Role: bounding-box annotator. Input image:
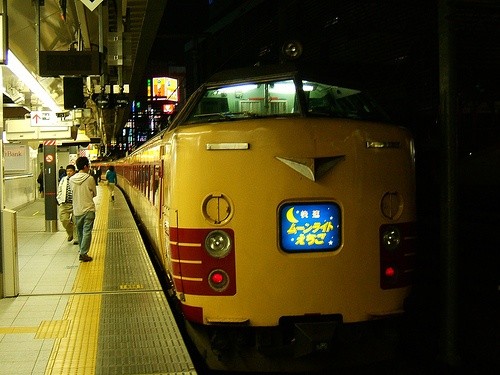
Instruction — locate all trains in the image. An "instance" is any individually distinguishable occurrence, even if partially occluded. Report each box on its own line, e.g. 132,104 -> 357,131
91,40 -> 418,361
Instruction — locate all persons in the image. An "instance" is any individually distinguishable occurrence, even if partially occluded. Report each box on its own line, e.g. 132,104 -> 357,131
59,166 -> 67,181
87,165 -> 102,185
105,166 -> 117,201
57,165 -> 80,245
37,169 -> 44,193
70,157 -> 97,262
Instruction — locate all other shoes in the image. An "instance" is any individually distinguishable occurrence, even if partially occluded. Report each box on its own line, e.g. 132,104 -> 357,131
68,236 -> 73,241
78,254 -> 92,261
72,241 -> 79,245
112,196 -> 114,200
